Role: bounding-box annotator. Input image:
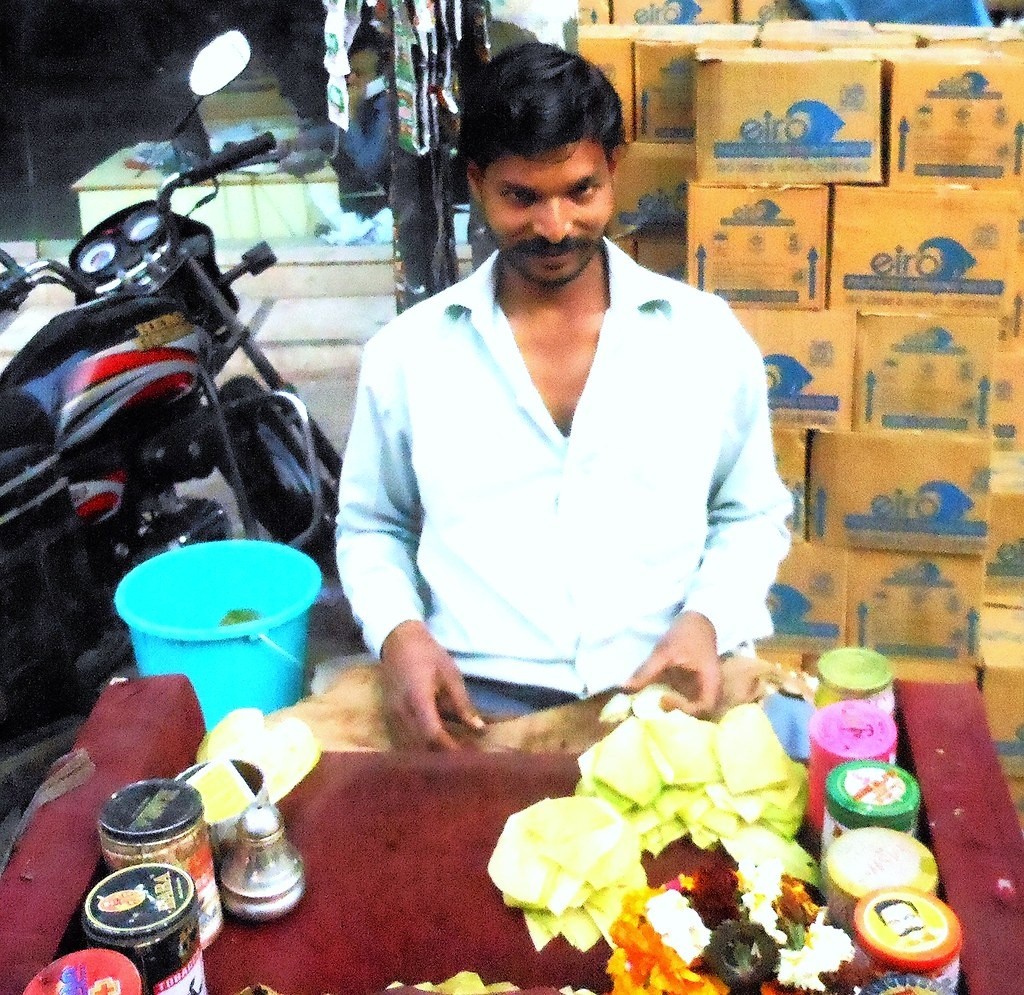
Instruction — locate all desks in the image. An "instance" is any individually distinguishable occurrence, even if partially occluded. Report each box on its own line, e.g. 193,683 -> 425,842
0,681 -> 1024,995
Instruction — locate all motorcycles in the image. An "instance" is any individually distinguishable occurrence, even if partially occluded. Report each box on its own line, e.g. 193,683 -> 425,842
0,25 -> 360,770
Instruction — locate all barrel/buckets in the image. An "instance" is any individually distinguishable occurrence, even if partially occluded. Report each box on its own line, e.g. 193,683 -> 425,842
112,539 -> 322,739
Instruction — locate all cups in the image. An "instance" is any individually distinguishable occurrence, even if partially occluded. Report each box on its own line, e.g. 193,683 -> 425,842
171,757 -> 270,871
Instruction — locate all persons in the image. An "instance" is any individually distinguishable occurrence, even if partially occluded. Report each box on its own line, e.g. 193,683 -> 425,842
334,42 -> 793,755
276,39 -> 390,185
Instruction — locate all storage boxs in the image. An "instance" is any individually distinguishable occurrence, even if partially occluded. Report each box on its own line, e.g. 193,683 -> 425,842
574,0 -> 1024,836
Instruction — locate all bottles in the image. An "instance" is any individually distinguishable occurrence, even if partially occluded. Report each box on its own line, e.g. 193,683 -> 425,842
805,645 -> 962,995
19,778 -> 222,995
